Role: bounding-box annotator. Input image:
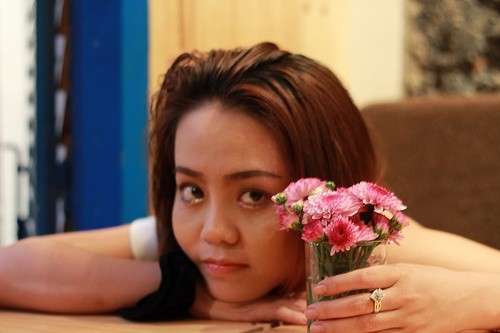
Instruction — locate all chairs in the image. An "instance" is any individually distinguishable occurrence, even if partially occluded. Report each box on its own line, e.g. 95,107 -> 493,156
359,94 -> 500,252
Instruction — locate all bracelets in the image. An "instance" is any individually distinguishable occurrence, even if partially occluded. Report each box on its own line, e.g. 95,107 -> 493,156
120,250 -> 196,322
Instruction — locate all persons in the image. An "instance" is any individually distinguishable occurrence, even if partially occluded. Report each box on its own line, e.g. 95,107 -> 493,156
0,42 -> 500,333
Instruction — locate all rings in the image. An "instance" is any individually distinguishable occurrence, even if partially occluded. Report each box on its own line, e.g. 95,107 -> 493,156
370,289 -> 385,314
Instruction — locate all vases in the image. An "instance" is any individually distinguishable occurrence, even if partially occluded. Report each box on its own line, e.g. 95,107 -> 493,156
306,236 -> 387,333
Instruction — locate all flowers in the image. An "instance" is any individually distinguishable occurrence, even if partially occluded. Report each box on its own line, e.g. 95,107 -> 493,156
271,177 -> 411,319
